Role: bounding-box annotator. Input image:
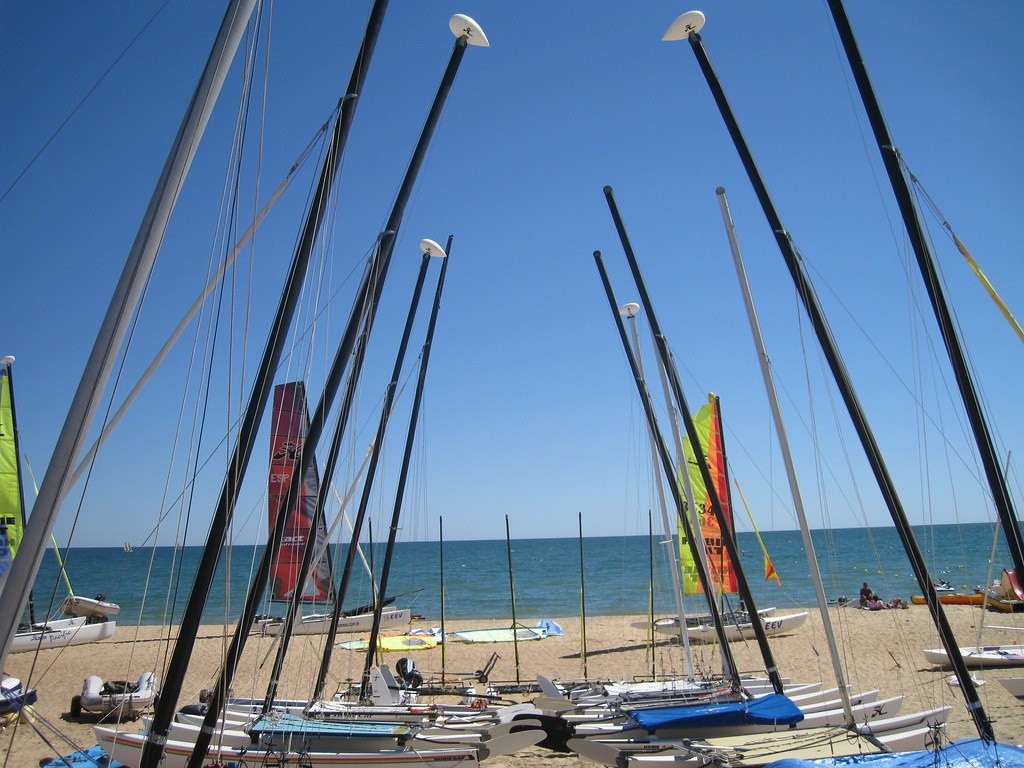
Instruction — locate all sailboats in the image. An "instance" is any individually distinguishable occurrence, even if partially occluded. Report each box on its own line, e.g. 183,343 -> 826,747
536,0 -> 1024,768
1,0 -> 566,768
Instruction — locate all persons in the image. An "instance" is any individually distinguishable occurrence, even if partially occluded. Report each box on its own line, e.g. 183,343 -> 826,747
860,583 -> 889,610
